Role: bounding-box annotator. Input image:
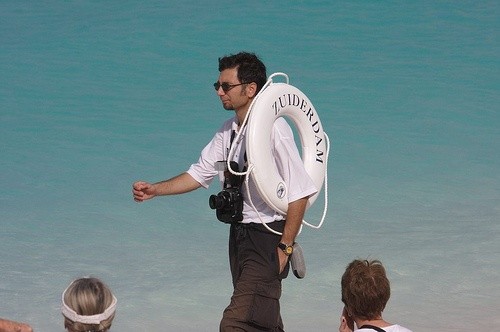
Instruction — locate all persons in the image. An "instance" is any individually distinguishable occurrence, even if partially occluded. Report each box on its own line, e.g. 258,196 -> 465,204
131,49 -> 319,331
337,257 -> 414,332
57,276 -> 117,332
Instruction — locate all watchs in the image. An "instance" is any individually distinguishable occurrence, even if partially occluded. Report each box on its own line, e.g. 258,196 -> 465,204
277,242 -> 294,256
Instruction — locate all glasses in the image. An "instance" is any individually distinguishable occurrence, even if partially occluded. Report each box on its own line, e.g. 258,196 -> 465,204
214,81 -> 252,92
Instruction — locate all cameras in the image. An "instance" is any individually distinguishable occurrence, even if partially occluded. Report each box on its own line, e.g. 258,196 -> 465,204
209,161 -> 243,223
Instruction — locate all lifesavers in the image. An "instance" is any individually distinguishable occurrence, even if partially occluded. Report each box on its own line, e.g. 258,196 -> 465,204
246,82 -> 326,217
